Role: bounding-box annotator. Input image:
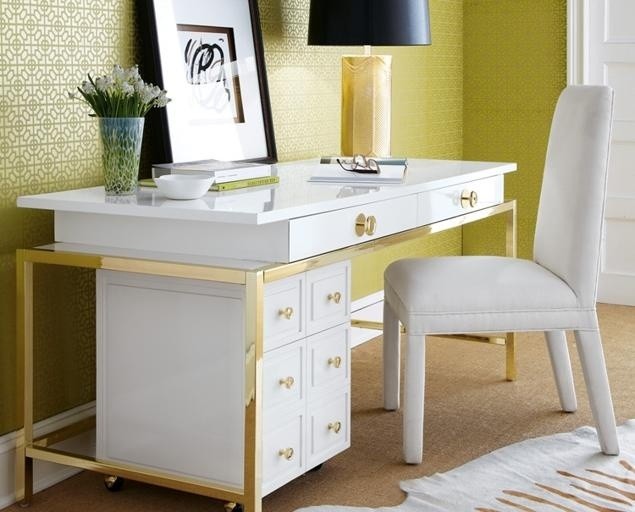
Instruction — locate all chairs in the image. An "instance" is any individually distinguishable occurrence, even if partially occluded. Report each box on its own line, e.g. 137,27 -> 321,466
382,84 -> 620,466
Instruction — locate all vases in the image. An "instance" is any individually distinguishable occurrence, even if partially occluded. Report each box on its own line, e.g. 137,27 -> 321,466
97,117 -> 146,196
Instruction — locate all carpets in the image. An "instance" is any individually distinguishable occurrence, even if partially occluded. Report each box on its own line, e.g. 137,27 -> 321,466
292,417 -> 635,512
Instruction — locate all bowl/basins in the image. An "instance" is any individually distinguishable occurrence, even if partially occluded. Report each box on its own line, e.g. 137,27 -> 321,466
153,174 -> 216,200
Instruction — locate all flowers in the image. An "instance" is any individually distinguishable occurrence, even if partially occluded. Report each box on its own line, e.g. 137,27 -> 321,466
68,63 -> 171,193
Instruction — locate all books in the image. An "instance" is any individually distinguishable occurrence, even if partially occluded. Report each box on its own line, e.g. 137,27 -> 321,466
308,157 -> 408,185
138,158 -> 280,191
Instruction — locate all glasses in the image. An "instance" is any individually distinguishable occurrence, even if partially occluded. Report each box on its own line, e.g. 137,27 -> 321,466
337,153 -> 380,173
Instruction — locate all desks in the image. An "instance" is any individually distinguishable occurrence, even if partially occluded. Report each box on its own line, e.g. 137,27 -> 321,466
14,158 -> 518,512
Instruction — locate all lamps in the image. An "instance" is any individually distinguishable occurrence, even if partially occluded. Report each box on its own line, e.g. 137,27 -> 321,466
306,0 -> 432,158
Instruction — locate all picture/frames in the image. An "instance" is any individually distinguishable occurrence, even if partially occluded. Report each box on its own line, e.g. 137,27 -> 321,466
135,0 -> 278,164
